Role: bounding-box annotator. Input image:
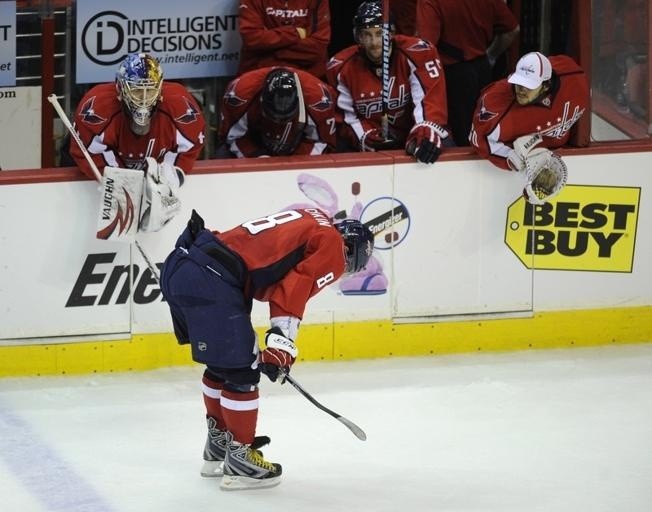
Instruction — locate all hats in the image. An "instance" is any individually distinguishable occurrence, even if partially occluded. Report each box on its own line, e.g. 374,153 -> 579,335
508,51 -> 552,90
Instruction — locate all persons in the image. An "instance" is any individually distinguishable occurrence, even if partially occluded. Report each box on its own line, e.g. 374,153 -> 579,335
323,1 -> 453,164
61,52 -> 207,232
159,207 -> 374,490
236,0 -> 331,78
468,52 -> 592,172
413,0 -> 521,147
215,65 -> 338,159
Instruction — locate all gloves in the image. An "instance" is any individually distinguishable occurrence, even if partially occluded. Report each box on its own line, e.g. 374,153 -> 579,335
405,121 -> 448,164
362,129 -> 402,152
259,333 -> 297,381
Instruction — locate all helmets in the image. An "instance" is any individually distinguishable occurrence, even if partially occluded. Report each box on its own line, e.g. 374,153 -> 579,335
334,219 -> 374,273
260,68 -> 300,121
352,2 -> 391,45
115,53 -> 164,137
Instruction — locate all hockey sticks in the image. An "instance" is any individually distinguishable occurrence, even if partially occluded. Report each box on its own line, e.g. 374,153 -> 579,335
279,71 -> 307,156
286,374 -> 366,440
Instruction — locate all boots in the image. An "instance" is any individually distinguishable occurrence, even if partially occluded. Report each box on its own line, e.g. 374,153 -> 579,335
220,432 -> 282,479
203,417 -> 262,462
